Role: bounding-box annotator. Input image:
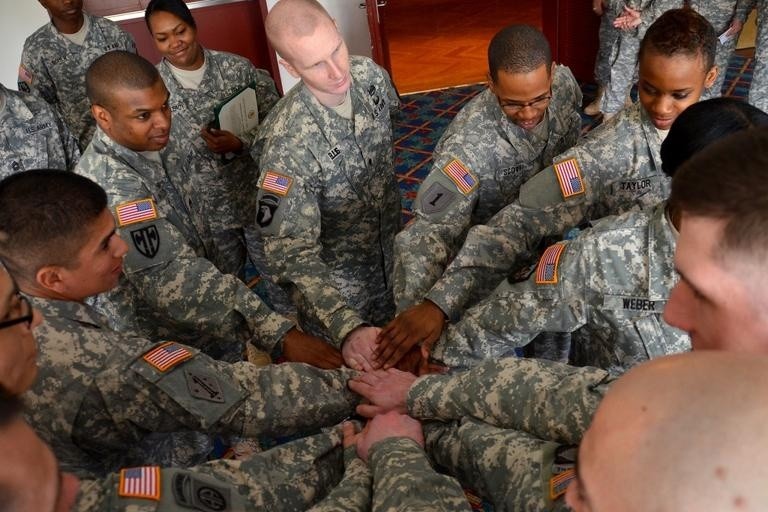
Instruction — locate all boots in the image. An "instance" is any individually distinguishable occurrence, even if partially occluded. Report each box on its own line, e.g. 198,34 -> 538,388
584,85 -> 633,123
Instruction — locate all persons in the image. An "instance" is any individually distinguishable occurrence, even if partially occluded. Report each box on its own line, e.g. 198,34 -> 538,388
0,0 -> 768,512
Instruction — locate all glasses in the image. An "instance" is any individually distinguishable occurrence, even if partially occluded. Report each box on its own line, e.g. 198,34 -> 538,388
0,258 -> 33,329
495,84 -> 552,110
